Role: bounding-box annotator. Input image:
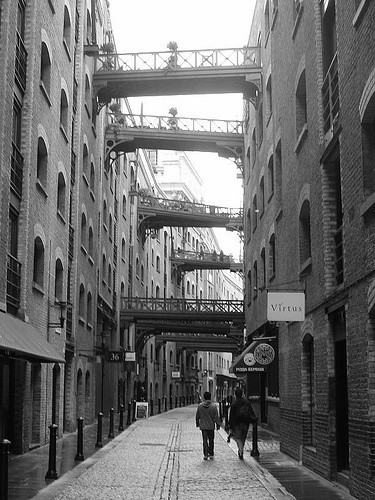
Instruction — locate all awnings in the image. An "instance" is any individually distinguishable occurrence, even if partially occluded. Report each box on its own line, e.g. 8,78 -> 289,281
229,336 -> 277,373
0,310 -> 65,363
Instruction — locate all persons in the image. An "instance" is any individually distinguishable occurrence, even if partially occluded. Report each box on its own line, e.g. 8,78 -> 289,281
229,388 -> 257,459
195,392 -> 219,460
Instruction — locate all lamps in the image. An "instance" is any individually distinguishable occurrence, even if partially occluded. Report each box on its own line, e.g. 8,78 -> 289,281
109,150 -> 118,160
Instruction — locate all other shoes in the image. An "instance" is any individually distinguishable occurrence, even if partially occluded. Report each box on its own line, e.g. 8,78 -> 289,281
239,451 -> 244,459
203,456 -> 208,460
210,456 -> 214,460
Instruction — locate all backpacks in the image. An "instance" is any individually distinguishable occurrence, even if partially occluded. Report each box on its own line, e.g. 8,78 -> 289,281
235,401 -> 251,422
